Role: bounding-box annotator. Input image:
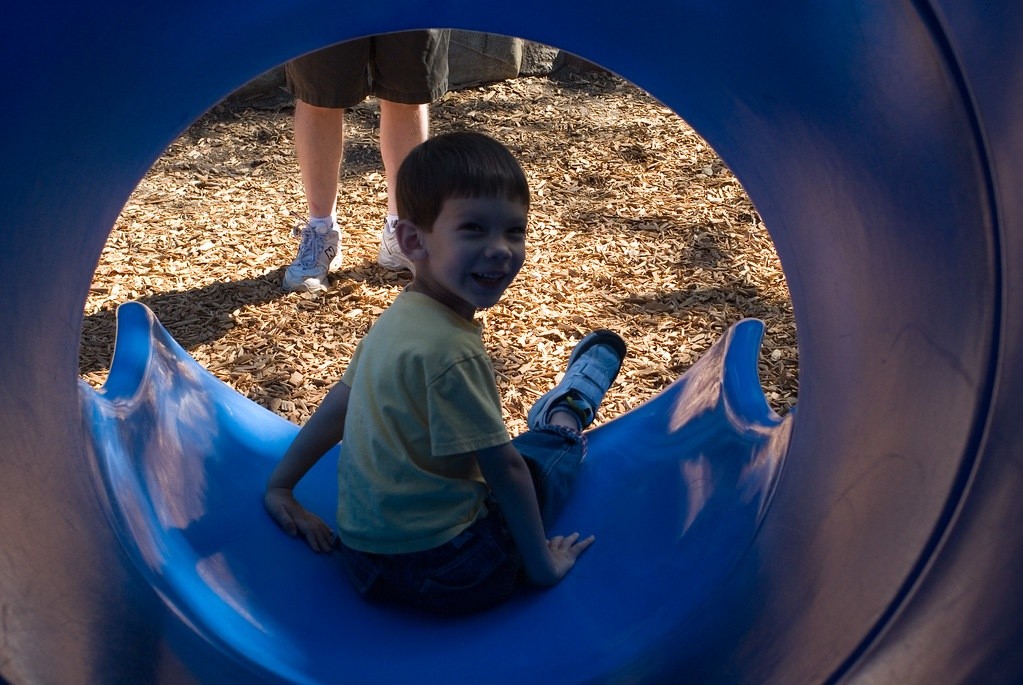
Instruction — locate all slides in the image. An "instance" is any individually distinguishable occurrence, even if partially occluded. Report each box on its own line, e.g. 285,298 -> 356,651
0,0 -> 1023,684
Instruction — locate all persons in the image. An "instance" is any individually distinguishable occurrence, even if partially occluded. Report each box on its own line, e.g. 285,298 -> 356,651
281,27 -> 451,296
263,132 -> 627,621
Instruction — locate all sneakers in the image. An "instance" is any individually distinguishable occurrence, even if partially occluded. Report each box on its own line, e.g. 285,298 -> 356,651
527,330 -> 626,432
378,218 -> 416,278
283,210 -> 342,293
